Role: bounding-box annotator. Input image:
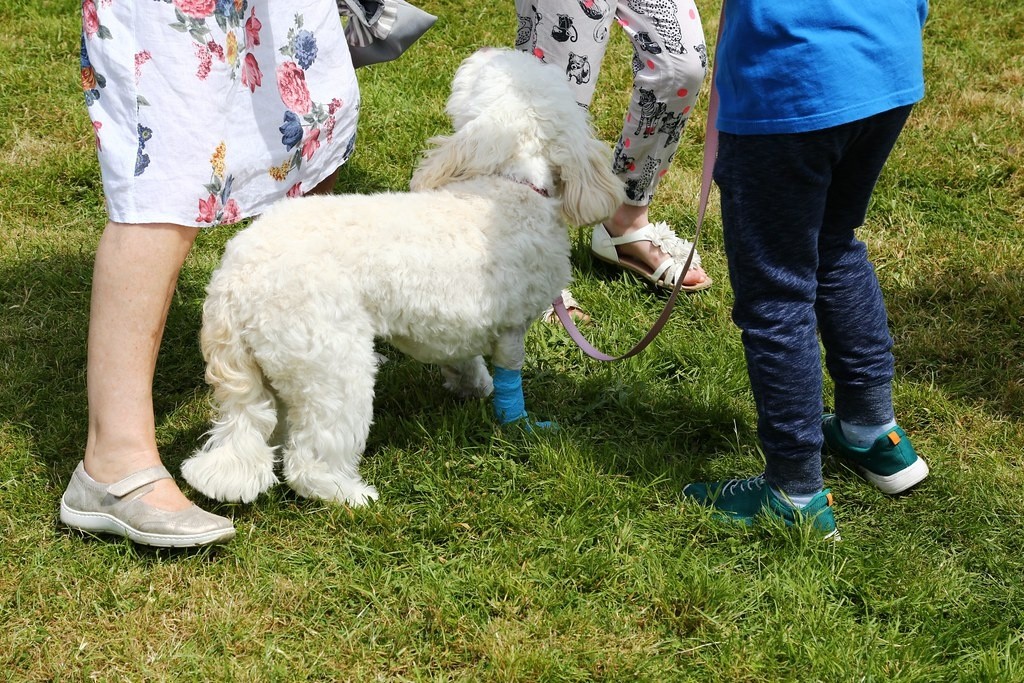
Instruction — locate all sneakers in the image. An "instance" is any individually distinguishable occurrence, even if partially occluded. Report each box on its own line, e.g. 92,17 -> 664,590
821,412 -> 929,493
682,477 -> 841,548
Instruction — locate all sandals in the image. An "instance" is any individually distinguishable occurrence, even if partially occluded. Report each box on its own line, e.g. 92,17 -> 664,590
590,214 -> 713,293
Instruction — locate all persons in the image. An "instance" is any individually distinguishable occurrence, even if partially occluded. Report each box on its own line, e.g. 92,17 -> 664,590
61,0 -> 361,548
515,0 -> 713,328
683,0 -> 930,544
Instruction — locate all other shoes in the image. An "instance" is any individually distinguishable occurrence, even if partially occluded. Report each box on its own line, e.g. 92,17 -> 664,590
59,462 -> 235,547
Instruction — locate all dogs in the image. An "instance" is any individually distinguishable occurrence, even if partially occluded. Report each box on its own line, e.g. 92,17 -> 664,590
181,48 -> 629,509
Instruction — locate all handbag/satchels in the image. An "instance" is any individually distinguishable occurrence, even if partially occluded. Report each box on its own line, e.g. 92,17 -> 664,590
341,0 -> 439,68
